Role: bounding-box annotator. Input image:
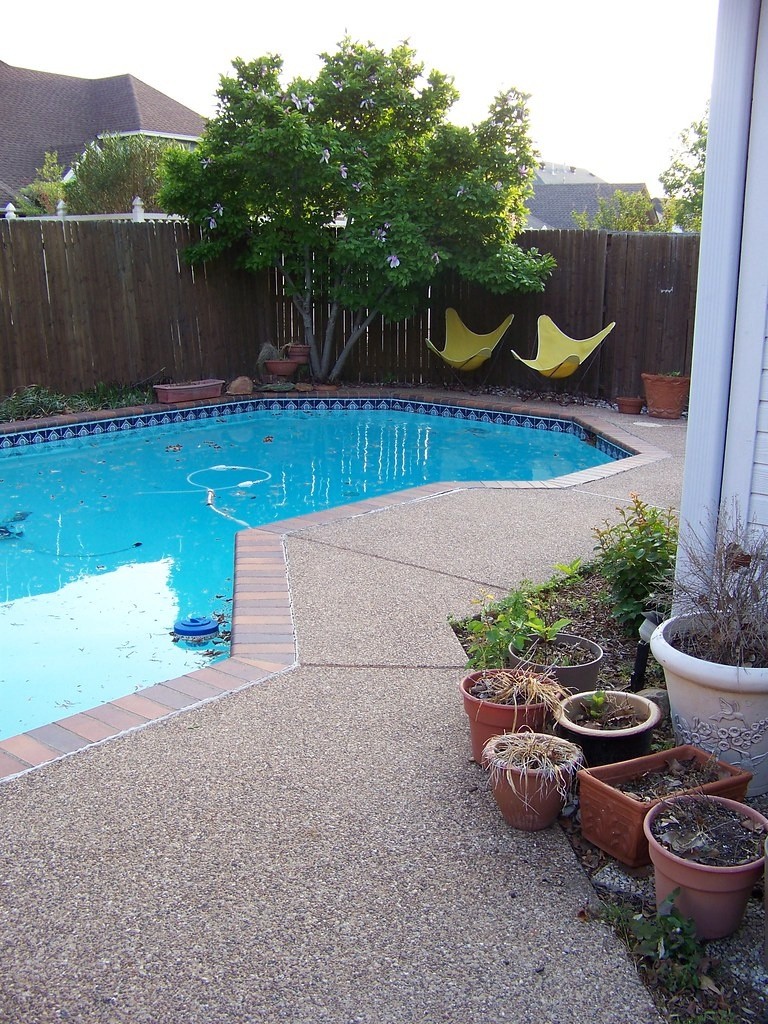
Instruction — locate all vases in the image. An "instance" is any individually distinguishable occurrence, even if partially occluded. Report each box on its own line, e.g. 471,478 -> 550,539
576,744 -> 752,868
483,732 -> 580,832
615,397 -> 645,414
264,360 -> 298,376
153,379 -> 225,404
642,795 -> 768,939
650,610 -> 768,798
459,668 -> 561,769
288,345 -> 312,364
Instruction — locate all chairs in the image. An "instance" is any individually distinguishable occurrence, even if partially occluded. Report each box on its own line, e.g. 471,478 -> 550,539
425,308 -> 514,396
510,315 -> 616,408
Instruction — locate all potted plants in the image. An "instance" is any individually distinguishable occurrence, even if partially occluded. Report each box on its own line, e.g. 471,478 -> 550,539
553,690 -> 662,768
509,626 -> 604,697
641,371 -> 689,419
